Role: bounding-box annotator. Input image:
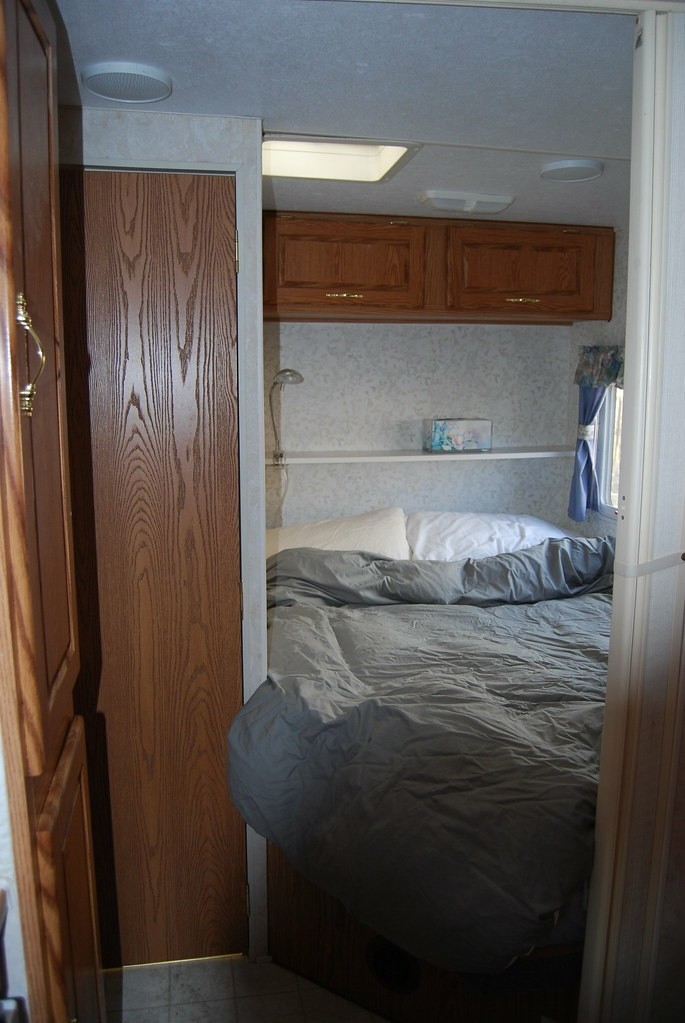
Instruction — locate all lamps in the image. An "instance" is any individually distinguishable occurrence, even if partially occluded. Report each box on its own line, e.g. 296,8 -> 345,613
268,368 -> 304,470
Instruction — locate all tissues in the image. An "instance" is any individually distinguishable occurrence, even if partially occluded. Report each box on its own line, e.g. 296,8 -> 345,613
421,415 -> 493,454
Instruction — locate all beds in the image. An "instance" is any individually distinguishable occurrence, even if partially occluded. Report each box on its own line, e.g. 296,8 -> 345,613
265,574 -> 612,991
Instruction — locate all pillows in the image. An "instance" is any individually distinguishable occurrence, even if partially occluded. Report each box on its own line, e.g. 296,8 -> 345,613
406,509 -> 568,563
266,505 -> 412,562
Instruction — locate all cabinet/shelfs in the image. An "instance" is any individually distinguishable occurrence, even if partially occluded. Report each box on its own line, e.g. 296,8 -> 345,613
262,207 -> 618,329
264,444 -> 578,469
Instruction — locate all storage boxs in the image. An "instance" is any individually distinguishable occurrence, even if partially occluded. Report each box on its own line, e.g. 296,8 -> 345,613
421,415 -> 494,455
